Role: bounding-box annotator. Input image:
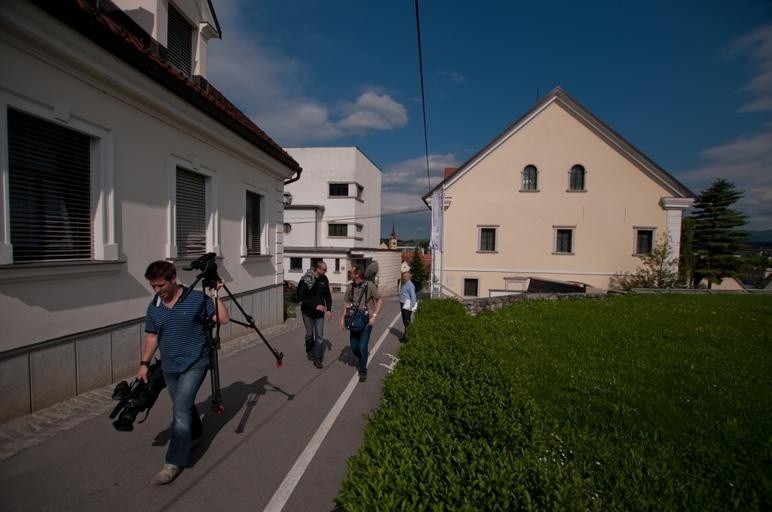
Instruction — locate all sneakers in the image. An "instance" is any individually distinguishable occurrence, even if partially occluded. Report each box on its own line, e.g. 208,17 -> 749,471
157,464 -> 182,483
315,359 -> 323,369
360,373 -> 366,381
307,351 -> 313,359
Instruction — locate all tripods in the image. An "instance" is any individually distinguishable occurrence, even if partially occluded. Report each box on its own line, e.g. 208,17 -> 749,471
200,279 -> 284,412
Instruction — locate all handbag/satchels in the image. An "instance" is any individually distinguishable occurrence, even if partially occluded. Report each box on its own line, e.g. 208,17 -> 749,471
344,307 -> 369,332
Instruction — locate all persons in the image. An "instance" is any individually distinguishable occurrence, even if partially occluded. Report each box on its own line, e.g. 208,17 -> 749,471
339,264 -> 383,381
398,271 -> 420,343
137,260 -> 231,486
298,260 -> 332,369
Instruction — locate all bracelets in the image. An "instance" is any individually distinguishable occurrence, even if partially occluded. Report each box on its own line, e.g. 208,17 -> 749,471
371,312 -> 378,317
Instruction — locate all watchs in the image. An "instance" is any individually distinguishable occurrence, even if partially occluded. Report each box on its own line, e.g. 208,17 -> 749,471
140,360 -> 151,370
